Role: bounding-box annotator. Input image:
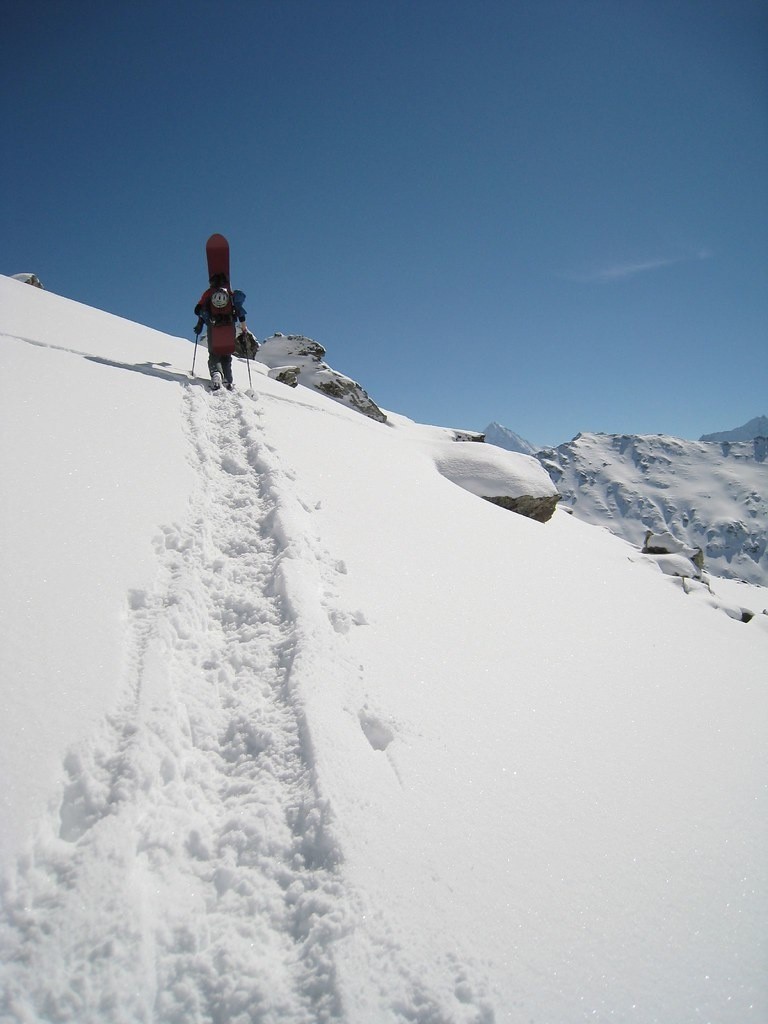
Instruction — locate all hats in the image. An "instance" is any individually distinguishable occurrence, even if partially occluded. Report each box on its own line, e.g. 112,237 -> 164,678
209,272 -> 228,282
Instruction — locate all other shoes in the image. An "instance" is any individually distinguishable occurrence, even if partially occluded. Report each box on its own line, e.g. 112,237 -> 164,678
210,372 -> 221,390
222,380 -> 233,391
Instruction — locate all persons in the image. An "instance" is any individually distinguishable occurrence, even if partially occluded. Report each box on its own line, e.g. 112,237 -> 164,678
193,272 -> 247,390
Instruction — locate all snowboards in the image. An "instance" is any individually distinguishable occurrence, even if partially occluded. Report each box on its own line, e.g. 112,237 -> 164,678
205,232 -> 236,356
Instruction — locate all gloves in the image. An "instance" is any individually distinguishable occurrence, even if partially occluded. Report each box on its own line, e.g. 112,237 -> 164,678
193,326 -> 202,335
